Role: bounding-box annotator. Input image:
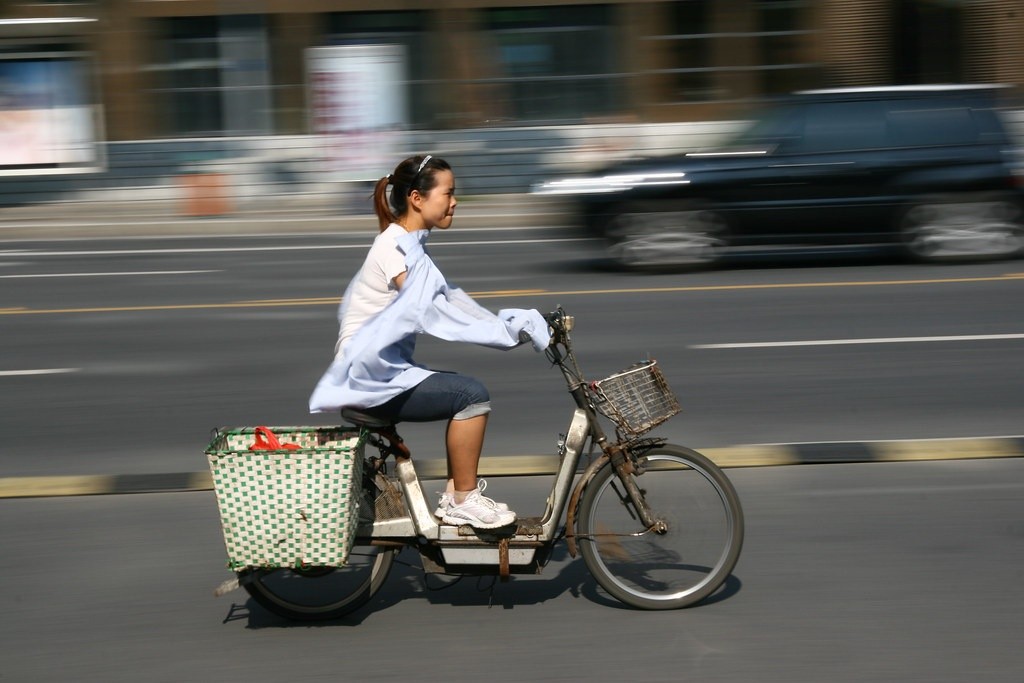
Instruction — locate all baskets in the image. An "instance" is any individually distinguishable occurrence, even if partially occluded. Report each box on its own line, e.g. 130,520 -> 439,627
203,427 -> 368,568
596,359 -> 682,434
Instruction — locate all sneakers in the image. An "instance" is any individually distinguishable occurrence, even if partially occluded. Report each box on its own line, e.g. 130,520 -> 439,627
434,490 -> 508,519
442,479 -> 517,529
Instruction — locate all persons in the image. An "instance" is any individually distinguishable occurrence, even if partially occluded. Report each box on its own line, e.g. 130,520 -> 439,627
335,155 -> 550,530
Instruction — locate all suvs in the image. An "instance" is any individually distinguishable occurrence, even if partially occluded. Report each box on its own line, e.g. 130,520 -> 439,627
531,83 -> 1023,273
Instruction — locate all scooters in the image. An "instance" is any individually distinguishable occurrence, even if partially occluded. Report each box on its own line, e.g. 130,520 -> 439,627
203,301 -> 745,619
